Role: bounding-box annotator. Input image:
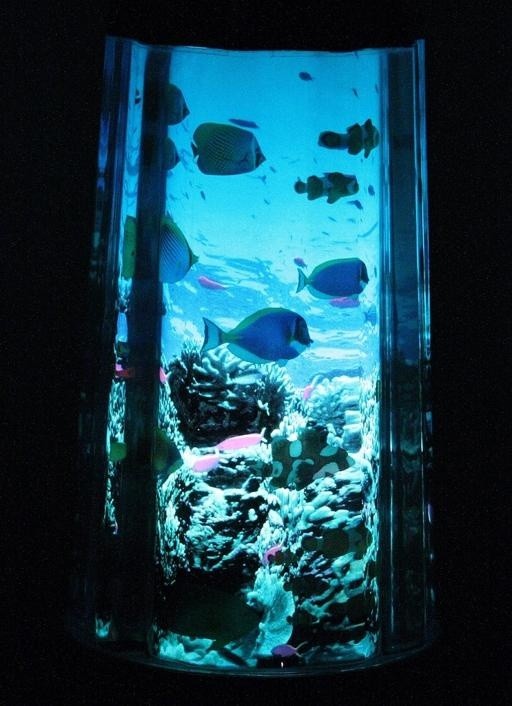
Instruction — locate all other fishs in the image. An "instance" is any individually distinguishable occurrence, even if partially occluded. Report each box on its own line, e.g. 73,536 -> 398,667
109,49 -> 383,473
